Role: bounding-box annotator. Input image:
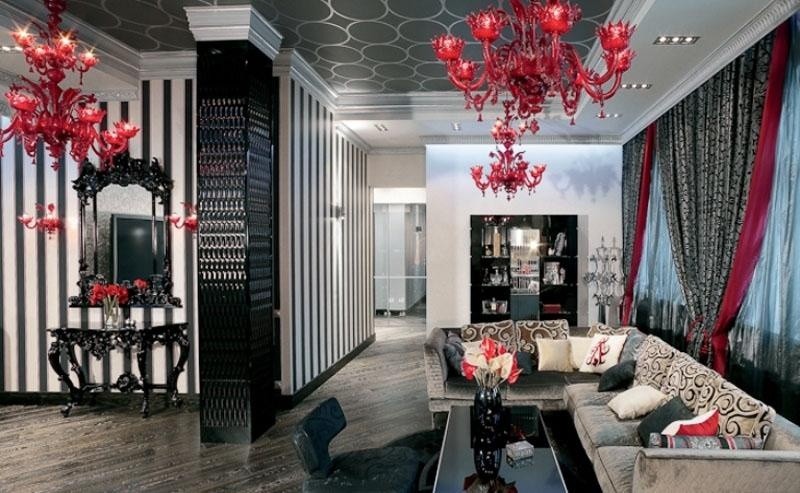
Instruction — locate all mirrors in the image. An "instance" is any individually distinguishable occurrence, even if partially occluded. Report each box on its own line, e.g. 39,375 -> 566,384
67,149 -> 183,307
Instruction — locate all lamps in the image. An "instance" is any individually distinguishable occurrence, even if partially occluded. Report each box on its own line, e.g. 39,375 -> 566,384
431,0 -> 637,125
470,93 -> 547,201
582,234 -> 618,324
169,201 -> 197,232
0,0 -> 140,171
17,202 -> 65,239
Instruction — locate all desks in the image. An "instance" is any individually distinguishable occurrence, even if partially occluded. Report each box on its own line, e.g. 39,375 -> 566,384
46,322 -> 191,418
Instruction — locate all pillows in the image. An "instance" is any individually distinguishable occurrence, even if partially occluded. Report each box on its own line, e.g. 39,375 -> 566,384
444,319 -> 628,376
598,334 -> 776,449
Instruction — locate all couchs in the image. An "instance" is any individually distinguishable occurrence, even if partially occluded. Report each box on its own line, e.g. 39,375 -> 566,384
422,326 -> 647,430
563,382 -> 800,493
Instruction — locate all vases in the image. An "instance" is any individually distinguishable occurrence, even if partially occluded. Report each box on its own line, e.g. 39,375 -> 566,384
474,386 -> 502,484
103,299 -> 119,330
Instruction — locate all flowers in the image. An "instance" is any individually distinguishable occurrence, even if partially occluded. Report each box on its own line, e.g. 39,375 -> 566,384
89,279 -> 146,322
460,333 -> 524,390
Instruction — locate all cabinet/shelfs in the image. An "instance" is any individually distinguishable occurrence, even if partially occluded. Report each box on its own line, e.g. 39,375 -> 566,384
471,215 -> 577,326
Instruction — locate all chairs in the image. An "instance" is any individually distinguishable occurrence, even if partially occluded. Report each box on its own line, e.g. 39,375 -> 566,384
290,398 -> 421,493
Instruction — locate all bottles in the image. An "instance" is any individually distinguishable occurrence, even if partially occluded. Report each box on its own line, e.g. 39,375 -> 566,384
484,267 -> 509,286
492,227 -> 501,257
